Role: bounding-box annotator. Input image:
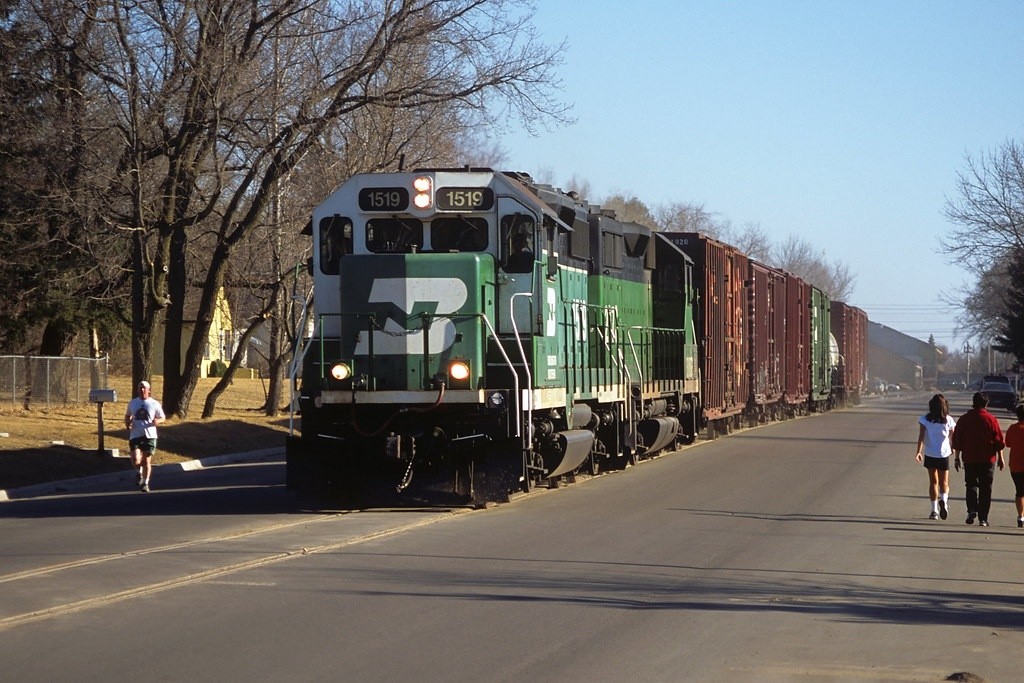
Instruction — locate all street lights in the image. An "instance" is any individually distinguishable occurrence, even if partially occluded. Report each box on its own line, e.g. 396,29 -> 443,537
988,334 -> 1007,373
916,365 -> 923,390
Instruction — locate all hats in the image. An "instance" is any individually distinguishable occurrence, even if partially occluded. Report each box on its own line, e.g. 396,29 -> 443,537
138,380 -> 151,388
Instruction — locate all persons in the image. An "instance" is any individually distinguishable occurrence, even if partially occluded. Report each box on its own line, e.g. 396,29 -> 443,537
125,380 -> 167,493
914,394 -> 957,520
951,391 -> 1006,527
1005,405 -> 1024,527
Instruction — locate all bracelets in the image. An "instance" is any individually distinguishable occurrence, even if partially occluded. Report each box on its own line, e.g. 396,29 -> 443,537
954,458 -> 959,461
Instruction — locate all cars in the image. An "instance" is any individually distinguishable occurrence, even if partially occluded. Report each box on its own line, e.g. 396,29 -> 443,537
867,378 -> 900,395
966,379 -> 982,392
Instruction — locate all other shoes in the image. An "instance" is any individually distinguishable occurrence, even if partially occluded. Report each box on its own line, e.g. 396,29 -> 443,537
965,511 -> 976,524
135,471 -> 143,485
928,512 -> 939,520
142,484 -> 149,493
938,500 -> 948,520
1017,516 -> 1024,527
979,520 -> 990,527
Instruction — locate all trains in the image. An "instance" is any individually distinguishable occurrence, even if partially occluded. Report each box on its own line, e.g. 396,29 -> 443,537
285,168 -> 868,509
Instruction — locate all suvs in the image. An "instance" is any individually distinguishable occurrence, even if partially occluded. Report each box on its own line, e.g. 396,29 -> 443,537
936,378 -> 966,392
979,374 -> 1017,410
